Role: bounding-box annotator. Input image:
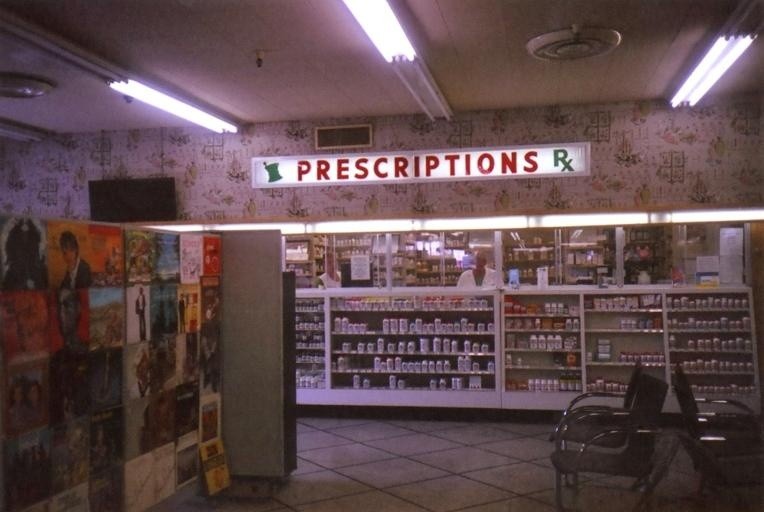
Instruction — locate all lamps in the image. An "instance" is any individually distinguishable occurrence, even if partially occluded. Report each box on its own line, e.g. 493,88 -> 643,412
108,69 -> 240,140
336,0 -> 419,64
669,2 -> 762,112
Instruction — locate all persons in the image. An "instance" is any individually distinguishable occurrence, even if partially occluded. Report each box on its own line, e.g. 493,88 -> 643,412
456,249 -> 504,288
26,378 -> 46,426
129,348 -> 151,399
7,383 -> 35,430
313,251 -> 342,289
53,287 -> 89,360
59,230 -> 92,290
179,293 -> 186,334
134,287 -> 148,344
623,240 -> 657,286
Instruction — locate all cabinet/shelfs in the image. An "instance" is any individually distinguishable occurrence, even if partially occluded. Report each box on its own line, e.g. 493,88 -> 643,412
274,222 -> 762,418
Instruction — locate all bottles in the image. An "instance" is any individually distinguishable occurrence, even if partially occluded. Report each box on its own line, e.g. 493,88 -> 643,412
335,237 -> 371,258
589,296 -> 663,310
332,337 -> 491,352
691,381 -> 755,392
470,375 -> 481,389
537,268 -> 549,287
617,351 -> 665,361
505,295 -> 581,314
505,352 -> 525,367
350,374 -> 370,388
429,378 -> 464,391
667,294 -> 749,308
504,317 -> 579,329
670,334 -> 750,347
670,359 -> 755,372
330,316 -> 495,333
385,375 -> 405,389
507,333 -> 580,349
618,317 -> 664,329
330,297 -> 494,311
587,375 -> 629,391
506,373 -> 583,392
332,354 -> 494,370
667,314 -> 752,330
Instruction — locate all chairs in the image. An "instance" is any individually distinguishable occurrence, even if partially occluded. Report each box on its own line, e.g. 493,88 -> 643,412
549,355 -> 763,512
